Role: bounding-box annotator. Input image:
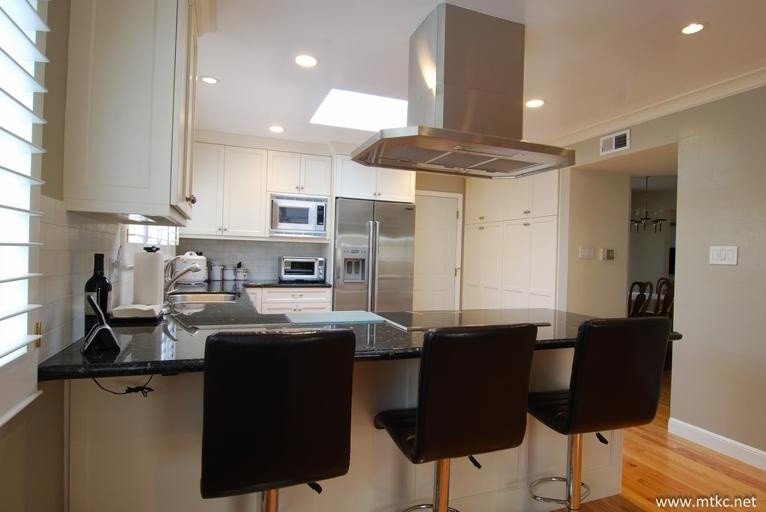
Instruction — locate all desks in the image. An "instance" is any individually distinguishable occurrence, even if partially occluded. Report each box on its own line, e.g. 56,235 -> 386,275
629,291 -> 665,314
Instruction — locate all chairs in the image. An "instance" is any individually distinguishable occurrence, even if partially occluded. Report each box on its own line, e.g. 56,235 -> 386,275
629,281 -> 653,317
654,277 -> 674,319
526,316 -> 674,511
199,329 -> 356,511
372,323 -> 538,510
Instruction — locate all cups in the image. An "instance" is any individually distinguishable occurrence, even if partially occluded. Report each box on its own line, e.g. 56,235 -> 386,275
209,281 -> 244,293
209,264 -> 249,281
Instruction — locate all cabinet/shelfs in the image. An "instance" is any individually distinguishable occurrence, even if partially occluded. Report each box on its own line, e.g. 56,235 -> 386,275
246,286 -> 334,315
61,0 -> 417,244
463,169 -> 559,311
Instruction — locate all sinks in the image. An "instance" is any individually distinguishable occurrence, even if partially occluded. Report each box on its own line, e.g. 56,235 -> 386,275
169,292 -> 240,304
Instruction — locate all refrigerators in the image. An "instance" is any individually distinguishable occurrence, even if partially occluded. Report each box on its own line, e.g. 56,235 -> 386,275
334,197 -> 415,313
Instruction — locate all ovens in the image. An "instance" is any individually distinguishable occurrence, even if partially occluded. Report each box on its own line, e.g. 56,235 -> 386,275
278,256 -> 326,282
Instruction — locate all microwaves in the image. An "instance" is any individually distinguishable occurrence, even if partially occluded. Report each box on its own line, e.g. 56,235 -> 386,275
272,198 -> 326,231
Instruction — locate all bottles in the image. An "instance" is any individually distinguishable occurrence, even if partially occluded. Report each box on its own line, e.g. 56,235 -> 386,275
85,253 -> 112,342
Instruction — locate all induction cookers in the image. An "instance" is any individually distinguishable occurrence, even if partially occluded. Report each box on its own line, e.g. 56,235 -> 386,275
370,309 -> 552,332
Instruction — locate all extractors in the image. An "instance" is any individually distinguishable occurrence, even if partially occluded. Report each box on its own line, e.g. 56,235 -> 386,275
351,3 -> 574,179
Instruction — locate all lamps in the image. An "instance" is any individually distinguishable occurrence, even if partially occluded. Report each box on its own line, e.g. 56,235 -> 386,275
626,176 -> 667,234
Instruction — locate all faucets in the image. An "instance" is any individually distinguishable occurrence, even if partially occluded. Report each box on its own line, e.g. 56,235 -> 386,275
168,262 -> 201,291
171,314 -> 199,337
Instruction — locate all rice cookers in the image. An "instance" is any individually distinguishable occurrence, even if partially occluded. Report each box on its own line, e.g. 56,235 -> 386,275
172,251 -> 208,285
